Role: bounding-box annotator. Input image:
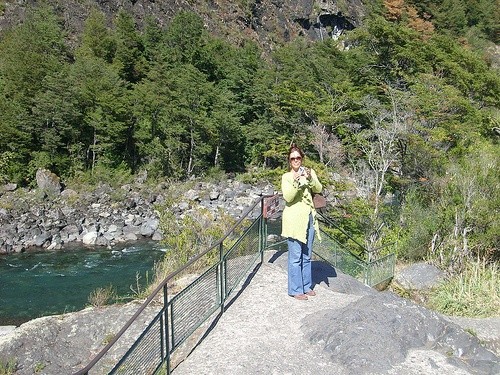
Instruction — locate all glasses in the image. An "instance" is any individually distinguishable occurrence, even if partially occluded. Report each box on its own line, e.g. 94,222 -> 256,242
290,156 -> 302,161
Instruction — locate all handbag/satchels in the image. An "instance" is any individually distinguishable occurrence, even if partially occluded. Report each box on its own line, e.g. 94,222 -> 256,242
307,166 -> 326,209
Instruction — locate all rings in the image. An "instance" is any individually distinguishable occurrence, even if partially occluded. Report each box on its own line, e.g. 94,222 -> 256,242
298,179 -> 299,180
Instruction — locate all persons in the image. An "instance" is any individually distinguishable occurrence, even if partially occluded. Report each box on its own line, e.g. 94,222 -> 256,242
281,147 -> 322,300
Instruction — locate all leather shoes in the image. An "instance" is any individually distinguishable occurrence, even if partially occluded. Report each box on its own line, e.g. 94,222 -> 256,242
289,294 -> 308,301
305,290 -> 316,297
